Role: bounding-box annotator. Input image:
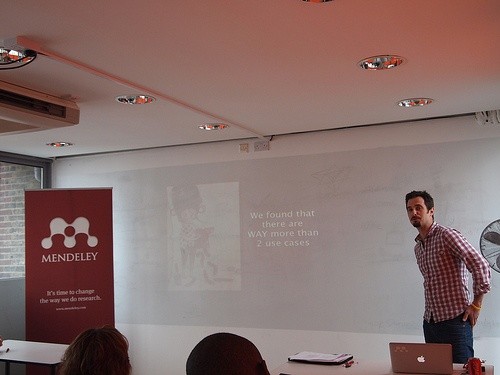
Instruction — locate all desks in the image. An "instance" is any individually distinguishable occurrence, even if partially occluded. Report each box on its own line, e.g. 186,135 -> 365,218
0,339 -> 71,375
268,356 -> 494,375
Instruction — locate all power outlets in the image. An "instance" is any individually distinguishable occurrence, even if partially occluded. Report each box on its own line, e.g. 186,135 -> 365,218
254,141 -> 269,151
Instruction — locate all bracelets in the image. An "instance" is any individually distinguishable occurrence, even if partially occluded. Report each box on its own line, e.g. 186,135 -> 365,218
472,303 -> 482,311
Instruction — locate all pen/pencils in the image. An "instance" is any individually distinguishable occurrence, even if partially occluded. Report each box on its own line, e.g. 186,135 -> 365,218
346,360 -> 355,368
6,348 -> 10,352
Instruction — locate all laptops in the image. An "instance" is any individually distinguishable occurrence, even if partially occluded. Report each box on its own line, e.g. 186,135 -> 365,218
389,342 -> 456,375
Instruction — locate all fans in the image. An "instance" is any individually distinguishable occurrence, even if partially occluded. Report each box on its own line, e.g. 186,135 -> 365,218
480,219 -> 500,274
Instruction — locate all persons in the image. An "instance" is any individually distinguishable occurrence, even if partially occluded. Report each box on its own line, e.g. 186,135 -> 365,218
58,326 -> 133,375
405,191 -> 491,363
185,332 -> 270,375
